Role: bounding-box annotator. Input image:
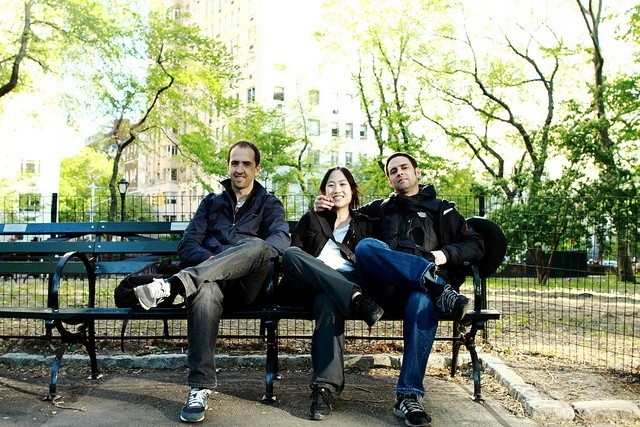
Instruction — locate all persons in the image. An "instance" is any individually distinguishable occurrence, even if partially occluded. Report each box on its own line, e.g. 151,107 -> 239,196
311,153 -> 484,427
132,140 -> 292,422
276,166 -> 384,420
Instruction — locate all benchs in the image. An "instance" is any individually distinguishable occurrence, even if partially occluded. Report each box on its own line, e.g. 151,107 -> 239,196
0,222 -> 95,397
48,220 -> 491,404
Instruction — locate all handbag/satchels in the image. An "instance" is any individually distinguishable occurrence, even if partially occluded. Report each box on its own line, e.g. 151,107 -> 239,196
114,258 -> 185,308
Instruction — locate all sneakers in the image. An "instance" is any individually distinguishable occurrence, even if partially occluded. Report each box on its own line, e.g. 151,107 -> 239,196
393,393 -> 431,427
133,277 -> 184,311
180,388 -> 211,422
352,293 -> 385,327
309,386 -> 334,420
434,284 -> 472,321
